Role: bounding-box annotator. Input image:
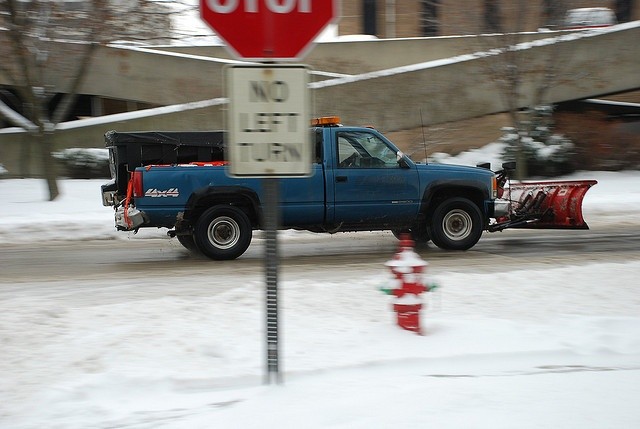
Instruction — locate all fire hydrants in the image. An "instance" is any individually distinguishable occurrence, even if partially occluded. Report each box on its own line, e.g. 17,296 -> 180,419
385,234 -> 429,333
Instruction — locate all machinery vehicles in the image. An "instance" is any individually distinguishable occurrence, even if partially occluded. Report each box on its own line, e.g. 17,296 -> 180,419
101,130 -> 596,236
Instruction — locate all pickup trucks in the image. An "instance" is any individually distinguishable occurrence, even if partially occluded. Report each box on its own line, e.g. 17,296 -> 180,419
116,116 -> 516,259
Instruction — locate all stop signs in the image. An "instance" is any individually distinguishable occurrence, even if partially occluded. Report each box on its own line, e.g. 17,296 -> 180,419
198,0 -> 342,63
